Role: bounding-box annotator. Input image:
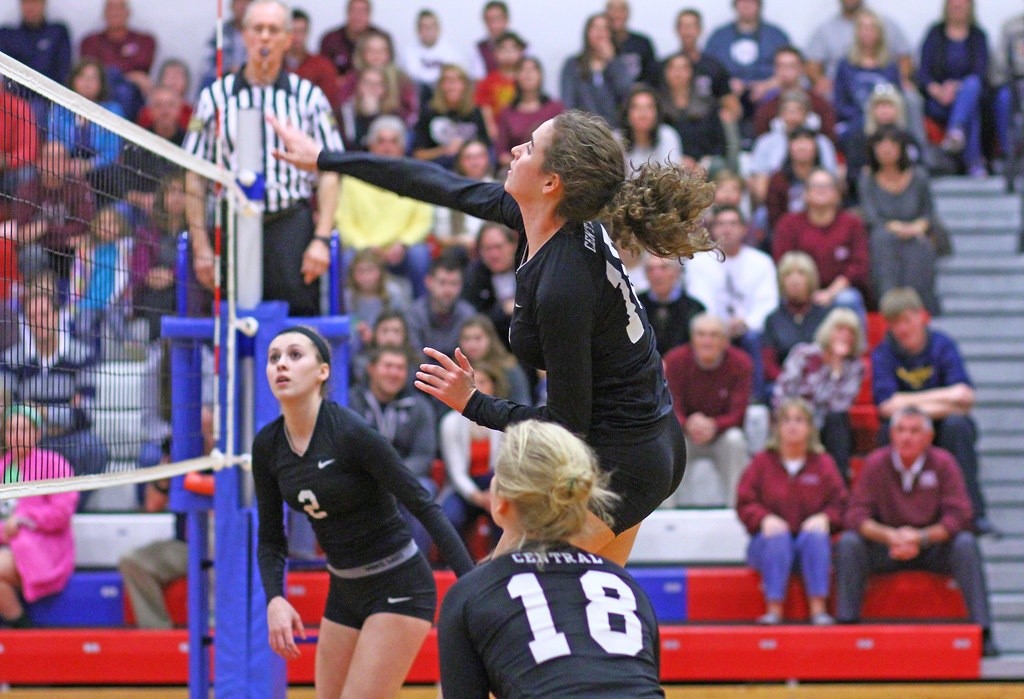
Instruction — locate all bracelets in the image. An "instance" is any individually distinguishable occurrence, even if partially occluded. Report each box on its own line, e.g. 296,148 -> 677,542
310,235 -> 330,247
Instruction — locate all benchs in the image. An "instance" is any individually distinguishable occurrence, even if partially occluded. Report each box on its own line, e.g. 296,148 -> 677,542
1,364 -> 983,683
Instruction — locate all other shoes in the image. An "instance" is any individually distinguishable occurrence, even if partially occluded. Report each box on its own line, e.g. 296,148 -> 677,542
974,515 -> 995,535
811,610 -> 836,626
756,612 -> 782,626
982,638 -> 998,656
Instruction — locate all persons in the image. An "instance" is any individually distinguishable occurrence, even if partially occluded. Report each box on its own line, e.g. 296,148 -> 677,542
0,0 -> 1024,654
252,324 -> 476,699
181,0 -> 349,317
438,420 -> 667,699
261,107 -> 687,573
